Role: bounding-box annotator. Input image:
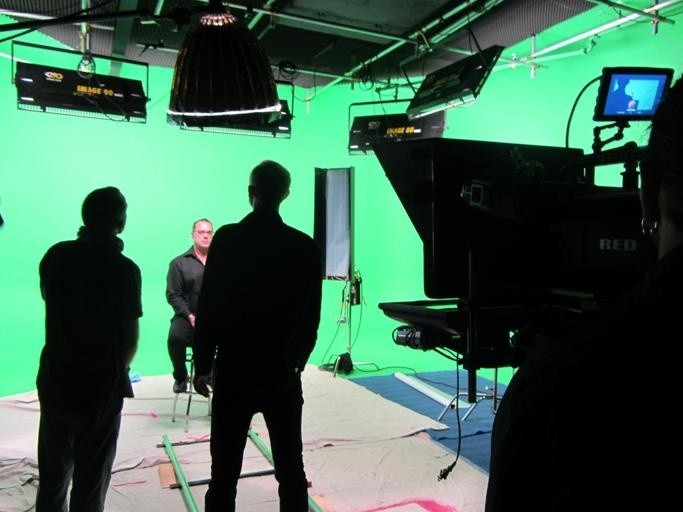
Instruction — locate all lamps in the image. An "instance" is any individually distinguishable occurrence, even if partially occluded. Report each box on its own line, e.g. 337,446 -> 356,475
166,0 -> 283,116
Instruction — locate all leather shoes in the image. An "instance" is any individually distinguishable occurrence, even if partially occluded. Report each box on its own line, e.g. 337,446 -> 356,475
193,377 -> 209,397
173,375 -> 189,393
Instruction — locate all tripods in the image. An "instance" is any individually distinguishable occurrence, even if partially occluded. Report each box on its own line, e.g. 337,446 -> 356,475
320,281 -> 380,377
438,368 -> 501,421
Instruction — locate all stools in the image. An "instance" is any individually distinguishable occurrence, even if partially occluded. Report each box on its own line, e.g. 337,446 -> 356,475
172,344 -> 220,423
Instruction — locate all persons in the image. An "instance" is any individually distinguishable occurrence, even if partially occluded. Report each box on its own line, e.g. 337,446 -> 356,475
484,75 -> 683,512
165,217 -> 217,393
34,185 -> 144,512
626,99 -> 637,112
189,157 -> 322,511
